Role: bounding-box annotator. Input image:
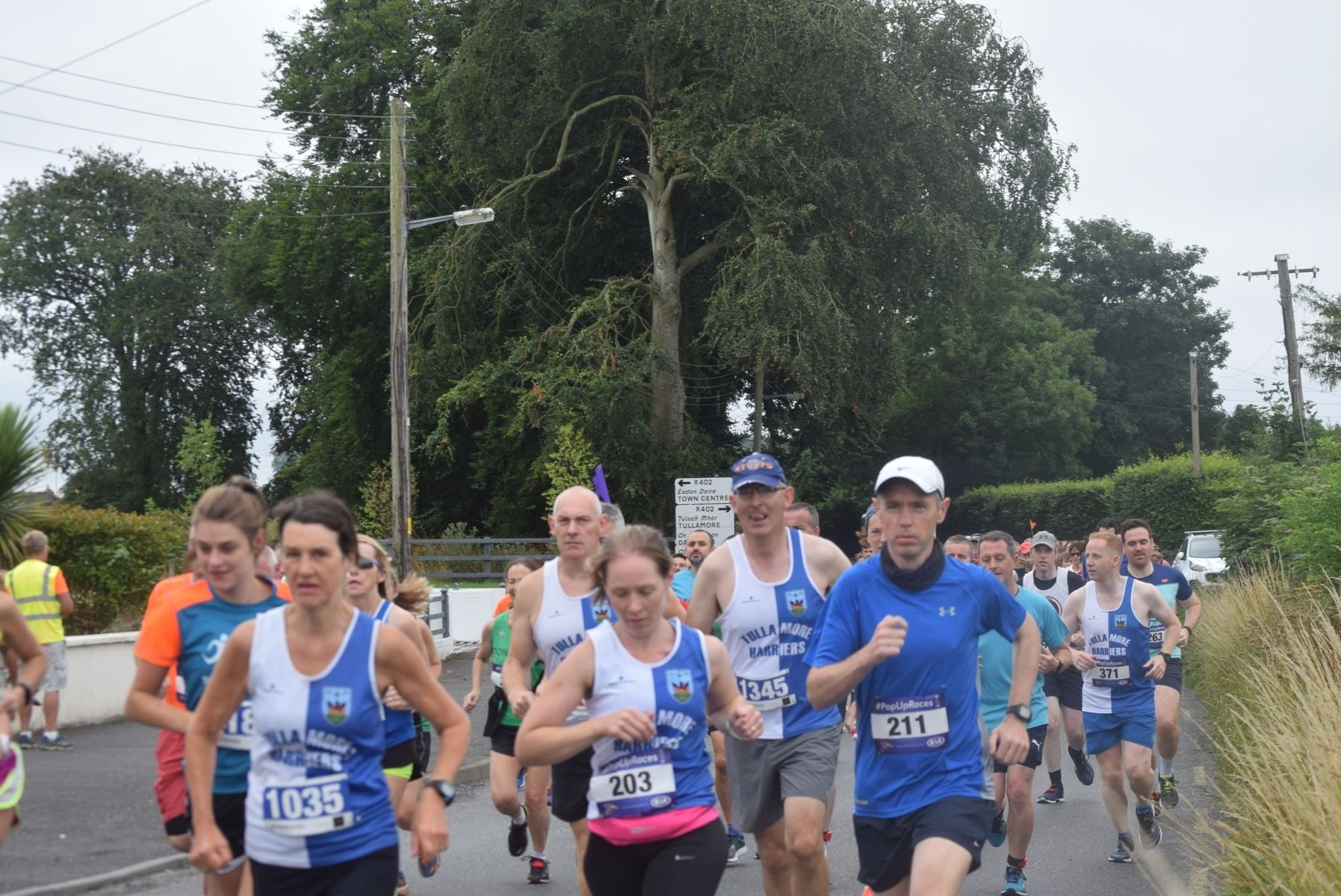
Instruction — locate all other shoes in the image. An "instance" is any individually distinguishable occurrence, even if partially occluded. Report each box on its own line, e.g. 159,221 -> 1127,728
392,873 -> 410,895
418,852 -> 439,876
727,833 -> 746,862
823,834 -> 831,848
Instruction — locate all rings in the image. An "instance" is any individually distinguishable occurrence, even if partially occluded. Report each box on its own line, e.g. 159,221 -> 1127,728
751,727 -> 756,733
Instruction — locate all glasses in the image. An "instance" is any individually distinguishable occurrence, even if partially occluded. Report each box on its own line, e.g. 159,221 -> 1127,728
1070,553 -> 1082,557
356,557 -> 383,571
735,486 -> 788,498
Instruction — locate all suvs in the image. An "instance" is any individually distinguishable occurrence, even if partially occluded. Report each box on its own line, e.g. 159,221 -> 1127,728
1172,530 -> 1229,592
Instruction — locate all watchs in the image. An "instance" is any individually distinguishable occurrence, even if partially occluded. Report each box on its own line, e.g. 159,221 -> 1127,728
1055,657 -> 1063,674
1158,652 -> 1171,663
424,779 -> 455,806
1005,704 -> 1033,724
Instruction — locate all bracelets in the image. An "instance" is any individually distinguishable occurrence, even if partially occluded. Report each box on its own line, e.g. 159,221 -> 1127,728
16,682 -> 31,706
1182,626 -> 1192,636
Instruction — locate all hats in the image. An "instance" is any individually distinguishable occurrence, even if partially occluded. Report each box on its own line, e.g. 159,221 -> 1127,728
1020,542 -> 1031,554
1029,531 -> 1057,549
873,454 -> 945,500
969,533 -> 983,544
729,453 -> 787,491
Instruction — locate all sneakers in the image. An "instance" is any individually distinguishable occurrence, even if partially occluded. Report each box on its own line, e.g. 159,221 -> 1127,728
1000,865 -> 1028,896
528,856 -> 550,883
39,736 -> 73,752
1108,834 -> 1136,862
18,733 -> 38,750
1151,790 -> 1162,818
985,805 -> 1008,848
509,806 -> 529,858
1157,770 -> 1179,808
1068,744 -> 1094,786
1135,802 -> 1161,849
1039,784 -> 1065,803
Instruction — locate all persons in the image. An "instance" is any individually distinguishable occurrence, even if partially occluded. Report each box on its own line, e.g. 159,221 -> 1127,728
0,452 -> 1201,896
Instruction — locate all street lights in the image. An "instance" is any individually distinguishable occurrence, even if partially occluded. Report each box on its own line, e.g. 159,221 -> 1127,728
390,209 -> 498,628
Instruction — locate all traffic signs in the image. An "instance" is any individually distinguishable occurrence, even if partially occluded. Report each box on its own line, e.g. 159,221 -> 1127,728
675,477 -> 732,504
676,506 -> 735,529
